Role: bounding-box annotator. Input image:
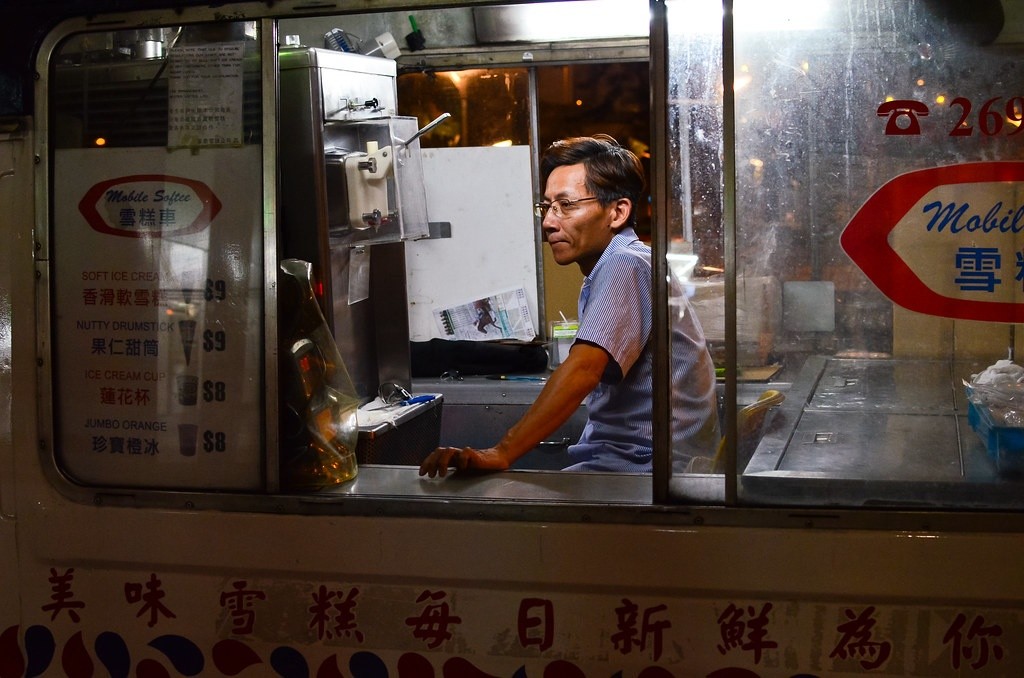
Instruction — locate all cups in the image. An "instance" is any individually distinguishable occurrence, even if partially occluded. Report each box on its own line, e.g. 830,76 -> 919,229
135,39 -> 162,59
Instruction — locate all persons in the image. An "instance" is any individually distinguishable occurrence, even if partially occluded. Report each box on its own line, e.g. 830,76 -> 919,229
418,135 -> 718,480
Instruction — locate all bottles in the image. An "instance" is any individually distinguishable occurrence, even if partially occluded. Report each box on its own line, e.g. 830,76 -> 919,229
348,32 -> 403,62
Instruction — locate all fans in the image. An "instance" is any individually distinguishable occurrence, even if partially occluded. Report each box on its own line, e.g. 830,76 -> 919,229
324,28 -> 402,60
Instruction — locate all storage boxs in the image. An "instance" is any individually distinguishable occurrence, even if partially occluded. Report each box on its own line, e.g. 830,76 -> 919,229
964,387 -> 1024,470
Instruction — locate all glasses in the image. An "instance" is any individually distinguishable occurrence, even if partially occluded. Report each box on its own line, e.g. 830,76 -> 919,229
534,195 -> 619,219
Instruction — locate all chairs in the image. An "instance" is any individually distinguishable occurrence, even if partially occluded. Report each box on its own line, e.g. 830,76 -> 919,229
708,391 -> 786,473
